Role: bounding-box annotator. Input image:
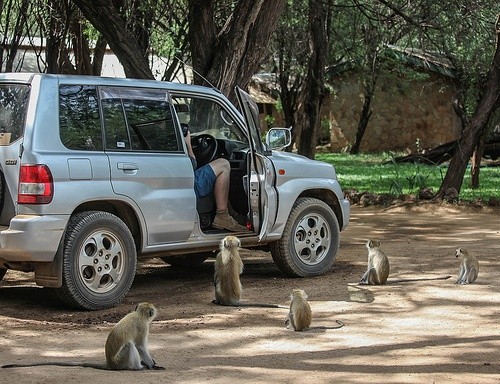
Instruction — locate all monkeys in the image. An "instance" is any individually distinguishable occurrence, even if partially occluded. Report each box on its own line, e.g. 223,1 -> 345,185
357,239 -> 452,286
283,287 -> 344,333
0,301 -> 166,372
455,246 -> 479,286
209,236 -> 291,309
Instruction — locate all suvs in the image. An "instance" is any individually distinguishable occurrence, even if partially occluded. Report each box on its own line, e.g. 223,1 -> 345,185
0,72 -> 352,310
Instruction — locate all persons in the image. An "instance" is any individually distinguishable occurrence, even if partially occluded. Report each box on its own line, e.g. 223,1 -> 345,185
183,130 -> 248,232
